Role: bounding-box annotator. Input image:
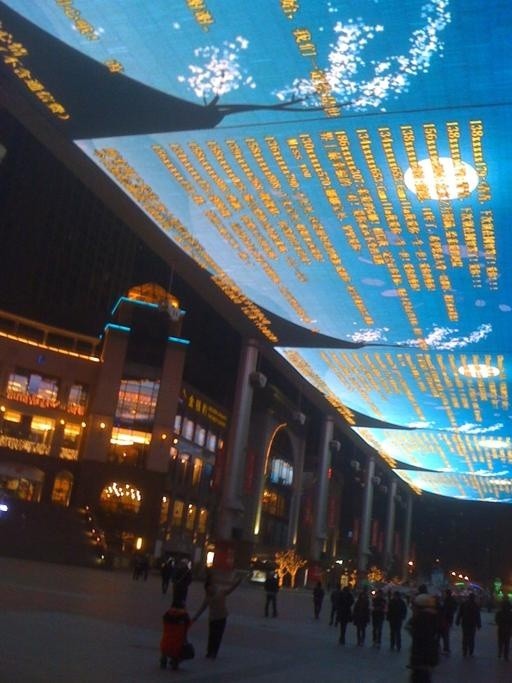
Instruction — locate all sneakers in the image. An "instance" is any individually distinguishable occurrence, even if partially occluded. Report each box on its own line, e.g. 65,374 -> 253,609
161,657 -> 178,669
206,653 -> 216,658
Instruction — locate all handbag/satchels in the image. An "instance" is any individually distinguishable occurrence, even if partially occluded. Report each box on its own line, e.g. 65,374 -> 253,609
181,642 -> 195,658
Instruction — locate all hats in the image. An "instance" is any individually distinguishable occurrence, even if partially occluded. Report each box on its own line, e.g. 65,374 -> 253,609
414,593 -> 432,608
